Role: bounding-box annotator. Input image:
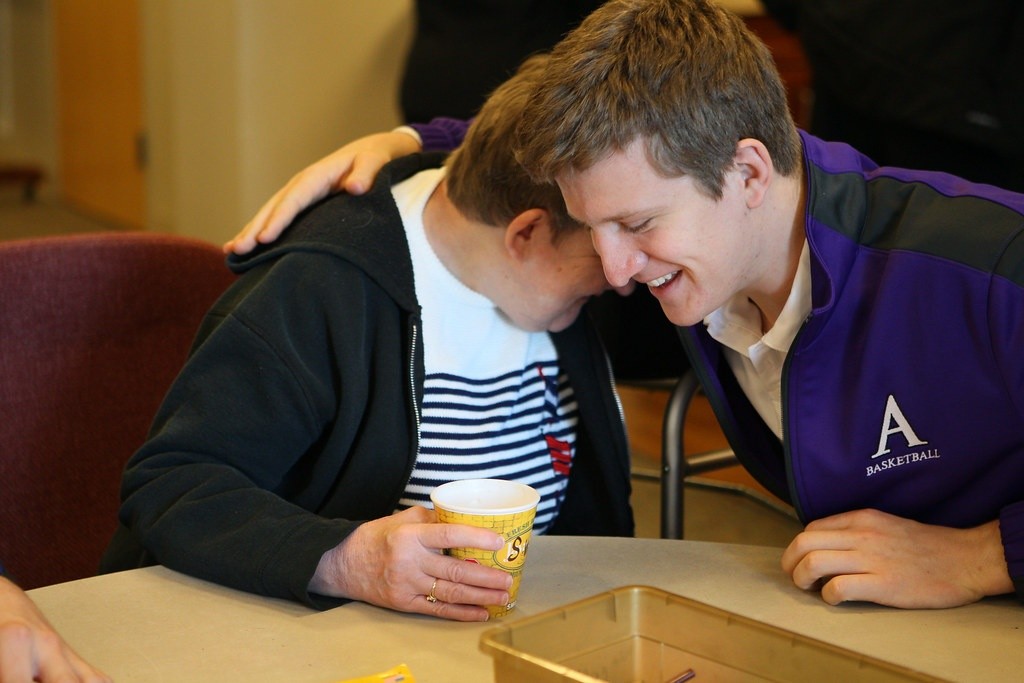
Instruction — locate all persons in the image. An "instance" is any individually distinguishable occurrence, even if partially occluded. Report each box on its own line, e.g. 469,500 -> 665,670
0,575 -> 111,683
100,56 -> 692,621
221,0 -> 1024,608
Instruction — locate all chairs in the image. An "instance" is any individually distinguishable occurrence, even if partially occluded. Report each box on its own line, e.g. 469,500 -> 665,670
0,230 -> 237,591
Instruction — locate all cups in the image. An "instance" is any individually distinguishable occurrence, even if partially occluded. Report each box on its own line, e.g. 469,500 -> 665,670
430,478 -> 539,619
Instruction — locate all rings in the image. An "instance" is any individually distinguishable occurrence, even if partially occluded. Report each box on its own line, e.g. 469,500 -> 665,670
427,578 -> 439,603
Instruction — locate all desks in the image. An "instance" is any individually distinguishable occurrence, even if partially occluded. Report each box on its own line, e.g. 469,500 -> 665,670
22,537 -> 1024,683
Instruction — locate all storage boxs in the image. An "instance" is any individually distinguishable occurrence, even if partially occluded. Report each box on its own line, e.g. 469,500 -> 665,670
478,586 -> 956,683
